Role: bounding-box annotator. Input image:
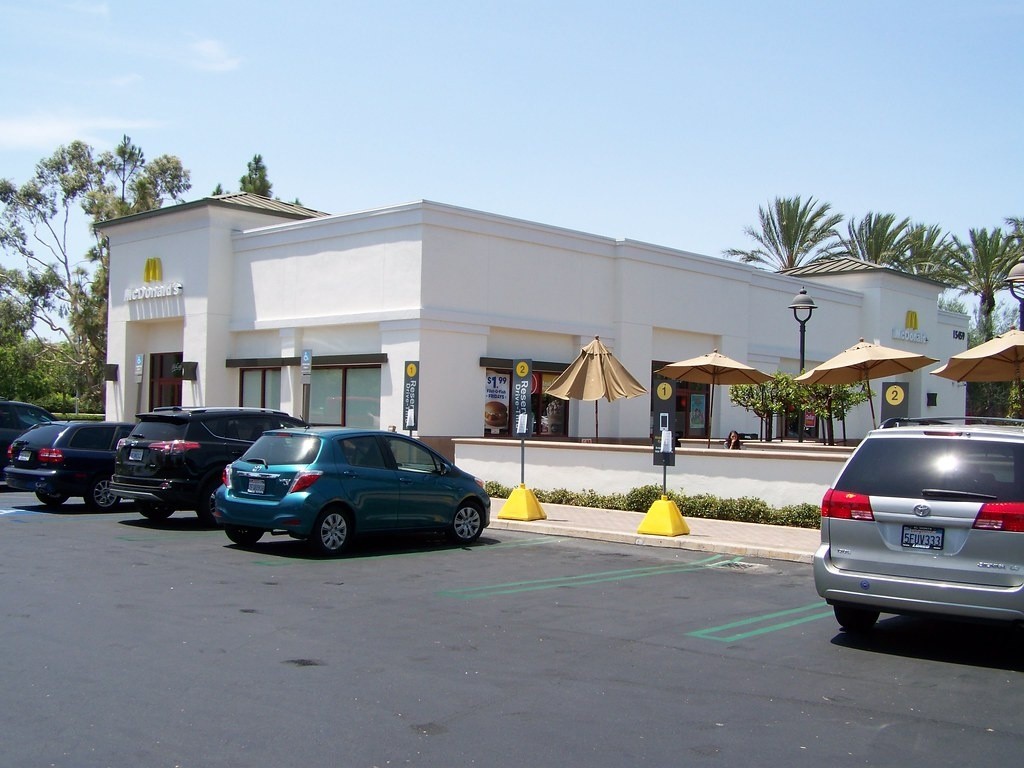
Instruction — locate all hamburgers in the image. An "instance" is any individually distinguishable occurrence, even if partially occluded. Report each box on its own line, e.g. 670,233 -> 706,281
485,401 -> 508,427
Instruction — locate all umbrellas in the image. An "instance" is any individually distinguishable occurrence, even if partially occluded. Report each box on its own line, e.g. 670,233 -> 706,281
544,335 -> 647,443
654,350 -> 777,448
931,324 -> 1024,421
795,337 -> 941,429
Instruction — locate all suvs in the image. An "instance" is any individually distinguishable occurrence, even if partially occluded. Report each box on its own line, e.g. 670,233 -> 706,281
4,421 -> 136,514
109,406 -> 309,526
0,397 -> 61,483
811,415 -> 1024,670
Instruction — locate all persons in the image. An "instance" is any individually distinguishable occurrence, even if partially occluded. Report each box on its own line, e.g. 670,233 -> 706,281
723,431 -> 740,449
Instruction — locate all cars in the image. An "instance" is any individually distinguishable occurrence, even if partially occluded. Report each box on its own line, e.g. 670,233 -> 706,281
211,425 -> 492,559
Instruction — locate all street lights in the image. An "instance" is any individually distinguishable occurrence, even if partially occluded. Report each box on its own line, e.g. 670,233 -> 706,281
787,285 -> 818,443
1001,255 -> 1024,332
